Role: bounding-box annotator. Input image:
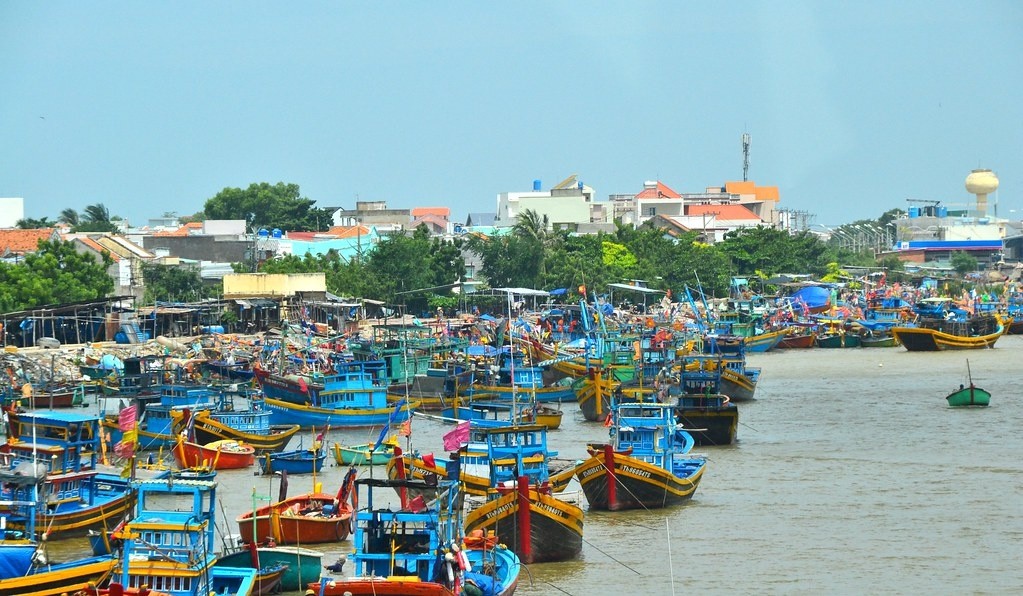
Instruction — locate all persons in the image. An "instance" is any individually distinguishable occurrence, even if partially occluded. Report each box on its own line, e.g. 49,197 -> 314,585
323,555 -> 346,573
477,308 -> 481,318
437,306 -> 443,322
107,368 -> 116,387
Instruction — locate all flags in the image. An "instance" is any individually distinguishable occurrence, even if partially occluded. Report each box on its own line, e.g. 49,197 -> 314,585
113,402 -> 140,497
443,420 -> 470,453
399,421 -> 410,437
298,377 -> 309,392
19,382 -> 32,398
410,454 -> 438,515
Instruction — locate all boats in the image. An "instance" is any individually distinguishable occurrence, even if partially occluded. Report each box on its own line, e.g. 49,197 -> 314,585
1,257 -> 1023,596
946,359 -> 992,407
